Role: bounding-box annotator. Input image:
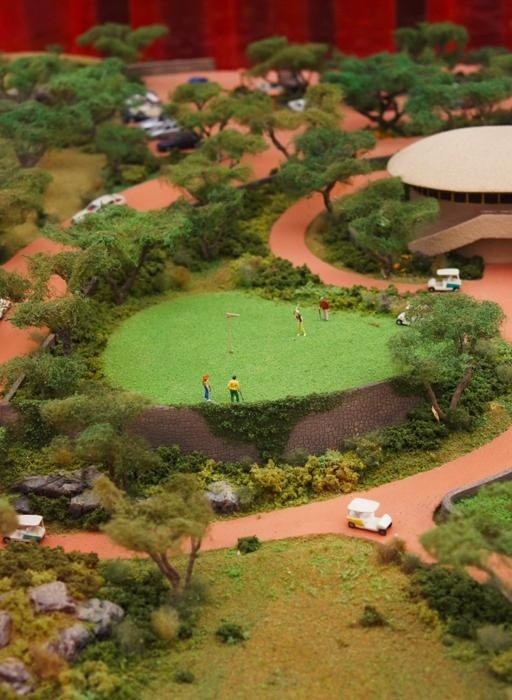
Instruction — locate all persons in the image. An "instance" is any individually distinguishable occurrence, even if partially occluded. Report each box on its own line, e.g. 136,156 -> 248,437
317,295 -> 331,321
223,374 -> 241,405
292,307 -> 308,338
201,372 -> 213,402
